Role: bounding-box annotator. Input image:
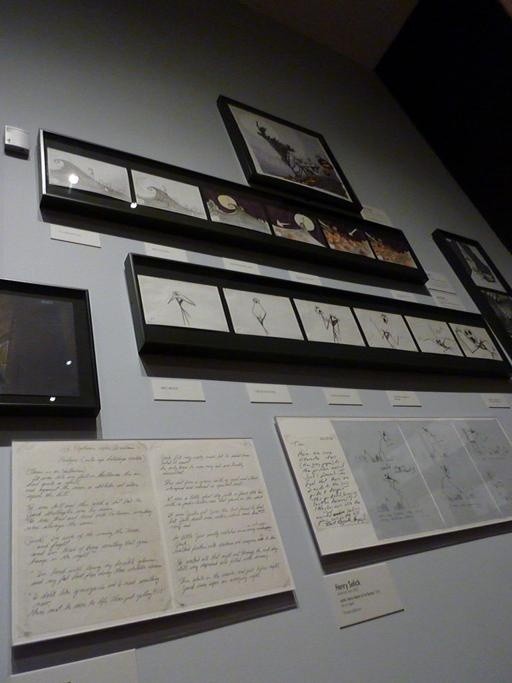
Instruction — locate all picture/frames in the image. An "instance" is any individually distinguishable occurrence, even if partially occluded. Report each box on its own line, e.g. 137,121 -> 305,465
35,127 -> 430,288
431,228 -> 512,357
0,277 -> 101,418
123,252 -> 512,382
215,94 -> 362,211
